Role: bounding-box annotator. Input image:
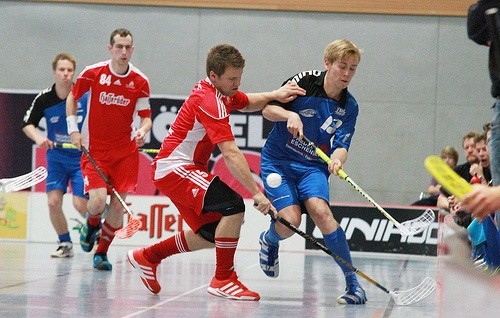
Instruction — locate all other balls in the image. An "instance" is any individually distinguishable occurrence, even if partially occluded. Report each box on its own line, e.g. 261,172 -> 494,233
266,171 -> 285,189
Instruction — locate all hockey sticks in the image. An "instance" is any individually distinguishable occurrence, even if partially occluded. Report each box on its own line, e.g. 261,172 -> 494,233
295,131 -> 436,237
53,141 -> 161,155
0,165 -> 49,194
253,200 -> 439,307
81,147 -> 142,240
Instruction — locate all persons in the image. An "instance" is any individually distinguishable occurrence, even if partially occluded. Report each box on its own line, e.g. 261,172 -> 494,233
65,29 -> 152,270
22,52 -> 108,258
126,44 -> 306,303
408,0 -> 500,318
257,40 -> 367,304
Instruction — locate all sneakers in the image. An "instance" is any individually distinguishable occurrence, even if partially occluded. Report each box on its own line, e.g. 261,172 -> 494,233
259,230 -> 280,278
207,272 -> 260,301
51,242 -> 75,258
127,248 -> 161,293
92,253 -> 113,271
80,213 -> 103,252
336,286 -> 367,305
